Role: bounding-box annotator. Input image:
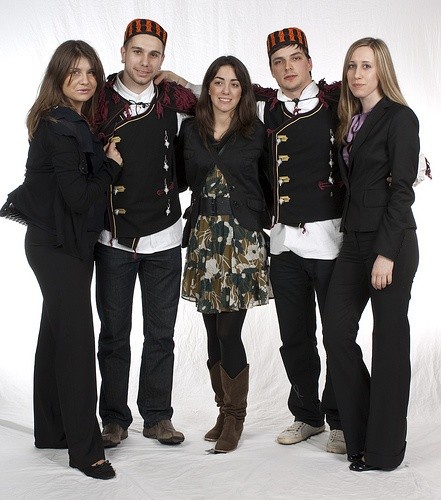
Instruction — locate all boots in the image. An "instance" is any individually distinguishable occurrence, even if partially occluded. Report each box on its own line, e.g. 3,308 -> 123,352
214,362 -> 249,452
205,360 -> 224,442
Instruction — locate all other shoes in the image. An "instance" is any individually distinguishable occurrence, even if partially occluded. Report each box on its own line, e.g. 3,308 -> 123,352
69,457 -> 116,480
350,460 -> 379,472
347,448 -> 365,462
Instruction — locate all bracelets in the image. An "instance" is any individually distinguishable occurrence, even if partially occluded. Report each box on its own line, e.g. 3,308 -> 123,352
175,75 -> 180,84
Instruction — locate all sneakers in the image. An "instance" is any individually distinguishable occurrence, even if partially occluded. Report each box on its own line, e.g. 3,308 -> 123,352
143,420 -> 185,443
277,420 -> 325,444
101,423 -> 128,447
327,430 -> 347,454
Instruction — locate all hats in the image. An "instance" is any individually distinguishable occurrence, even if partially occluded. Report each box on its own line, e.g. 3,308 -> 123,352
267,28 -> 308,59
124,19 -> 167,45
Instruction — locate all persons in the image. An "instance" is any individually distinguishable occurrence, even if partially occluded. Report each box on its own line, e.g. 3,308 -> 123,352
321,39 -> 419,472
154,28 -> 393,455
173,56 -> 273,454
8,41 -> 123,479
79,19 -> 200,448
